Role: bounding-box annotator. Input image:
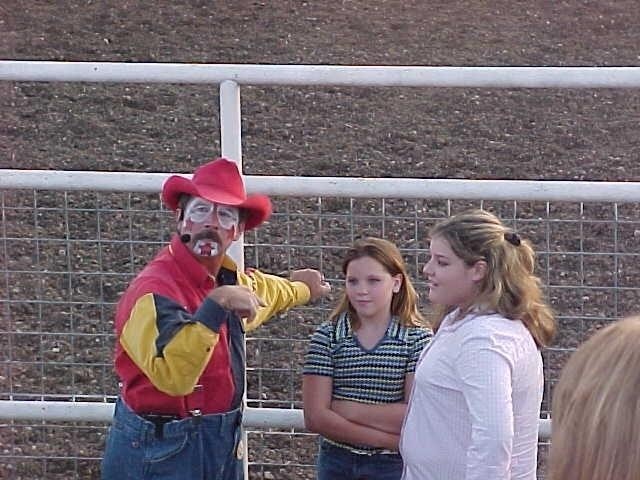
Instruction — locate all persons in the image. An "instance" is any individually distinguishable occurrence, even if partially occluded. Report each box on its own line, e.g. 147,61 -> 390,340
399,210 -> 558,479
98,157 -> 335,480
304,236 -> 433,480
549,314 -> 640,480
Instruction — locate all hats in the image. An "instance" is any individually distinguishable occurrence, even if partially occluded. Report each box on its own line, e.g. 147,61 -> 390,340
162,159 -> 273,233
